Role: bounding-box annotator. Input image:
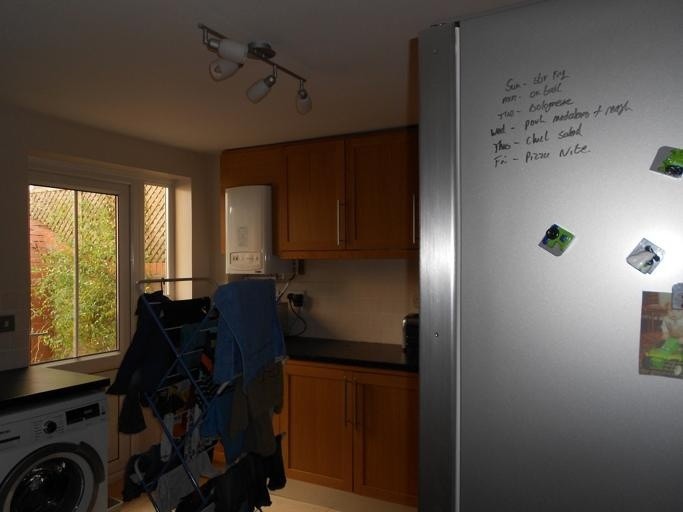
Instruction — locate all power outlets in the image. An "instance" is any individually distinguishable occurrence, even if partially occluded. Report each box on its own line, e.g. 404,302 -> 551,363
287,291 -> 304,311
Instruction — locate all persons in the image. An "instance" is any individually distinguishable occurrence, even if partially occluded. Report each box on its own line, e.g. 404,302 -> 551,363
655,291 -> 683,348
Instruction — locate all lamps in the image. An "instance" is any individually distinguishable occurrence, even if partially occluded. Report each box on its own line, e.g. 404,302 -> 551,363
199,24 -> 312,115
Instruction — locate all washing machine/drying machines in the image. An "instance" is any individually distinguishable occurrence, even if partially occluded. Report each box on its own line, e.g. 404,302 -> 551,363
0,391 -> 109,512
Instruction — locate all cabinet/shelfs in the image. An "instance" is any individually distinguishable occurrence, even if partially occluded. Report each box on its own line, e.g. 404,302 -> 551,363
219,124 -> 419,260
279,360 -> 419,508
